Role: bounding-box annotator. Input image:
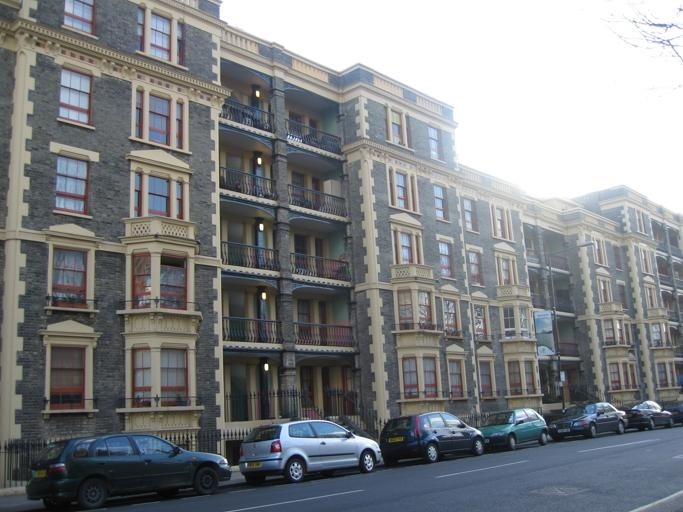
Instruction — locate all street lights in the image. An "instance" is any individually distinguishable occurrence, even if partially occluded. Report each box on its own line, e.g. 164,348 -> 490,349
547,240 -> 594,411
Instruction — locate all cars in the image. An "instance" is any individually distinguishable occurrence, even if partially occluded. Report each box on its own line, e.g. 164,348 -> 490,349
666,405 -> 683,426
548,401 -> 629,439
239,420 -> 384,482
623,400 -> 675,431
478,407 -> 548,450
380,411 -> 485,462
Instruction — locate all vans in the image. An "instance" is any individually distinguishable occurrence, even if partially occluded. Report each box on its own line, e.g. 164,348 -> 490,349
25,431 -> 232,509
